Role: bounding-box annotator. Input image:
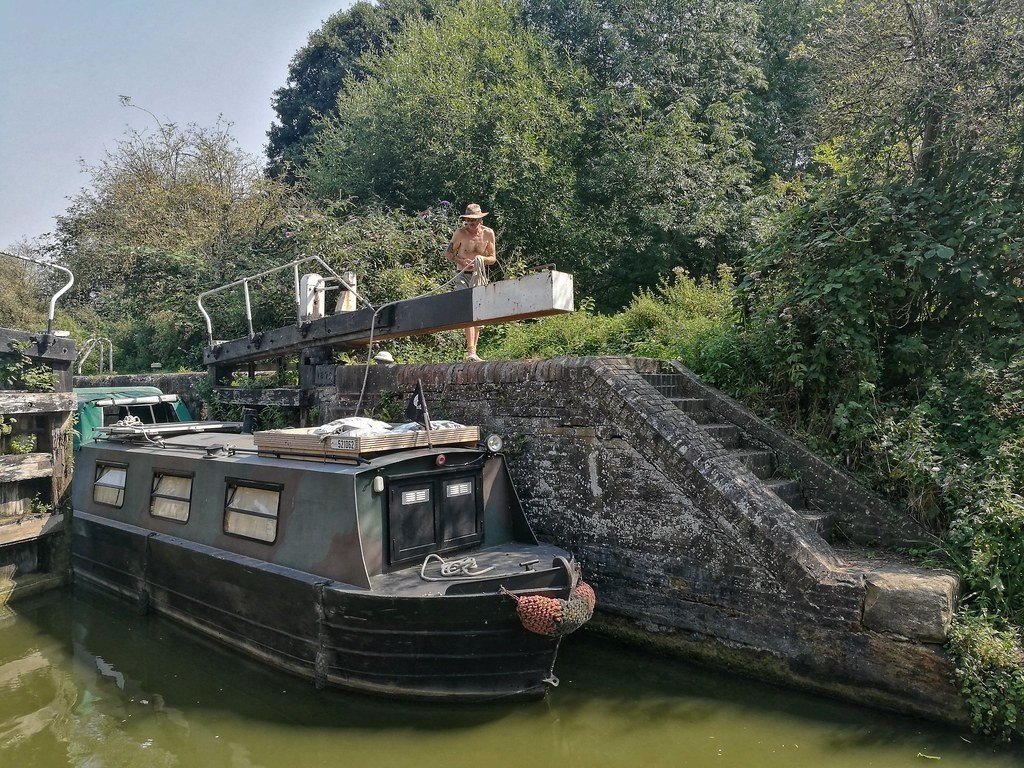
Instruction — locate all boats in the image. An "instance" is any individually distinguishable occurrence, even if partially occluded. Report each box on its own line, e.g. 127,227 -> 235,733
71,377 -> 600,702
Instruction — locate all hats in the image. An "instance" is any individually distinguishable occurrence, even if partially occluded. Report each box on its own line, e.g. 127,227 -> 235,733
459,203 -> 489,219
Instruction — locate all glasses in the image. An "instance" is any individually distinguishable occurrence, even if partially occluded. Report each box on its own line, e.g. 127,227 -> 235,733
465,217 -> 479,222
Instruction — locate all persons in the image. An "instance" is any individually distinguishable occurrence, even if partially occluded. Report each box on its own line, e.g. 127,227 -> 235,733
444,203 -> 495,363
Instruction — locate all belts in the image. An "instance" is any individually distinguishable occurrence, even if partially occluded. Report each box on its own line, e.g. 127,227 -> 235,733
456,270 -> 483,275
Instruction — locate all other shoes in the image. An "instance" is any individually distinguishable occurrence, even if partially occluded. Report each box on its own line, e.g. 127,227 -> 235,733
467,354 -> 487,362
